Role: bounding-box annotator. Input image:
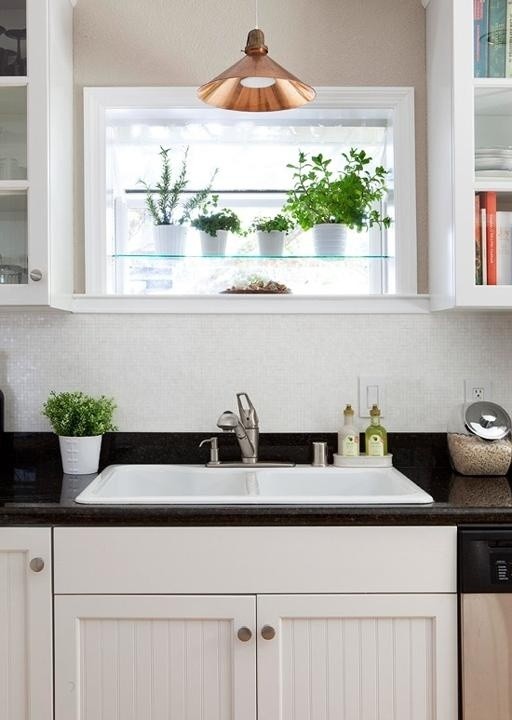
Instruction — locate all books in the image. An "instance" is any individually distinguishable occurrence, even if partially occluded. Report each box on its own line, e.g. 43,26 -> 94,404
475,191 -> 512,284
473,1 -> 512,78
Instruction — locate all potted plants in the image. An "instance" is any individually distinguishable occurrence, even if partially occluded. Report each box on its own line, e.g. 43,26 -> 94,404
143,147 -> 396,256
37,390 -> 120,476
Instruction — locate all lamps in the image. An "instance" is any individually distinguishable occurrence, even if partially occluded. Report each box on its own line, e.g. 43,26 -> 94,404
194,1 -> 318,113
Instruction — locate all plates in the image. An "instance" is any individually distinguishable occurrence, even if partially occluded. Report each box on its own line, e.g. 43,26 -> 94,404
475,145 -> 512,177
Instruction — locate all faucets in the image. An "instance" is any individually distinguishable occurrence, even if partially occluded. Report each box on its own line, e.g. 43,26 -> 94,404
213,391 -> 259,464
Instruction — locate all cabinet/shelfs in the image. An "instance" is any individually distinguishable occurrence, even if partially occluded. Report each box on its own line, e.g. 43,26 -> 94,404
57,524 -> 461,720
420,0 -> 512,315
0,522 -> 56,720
0,0 -> 63,310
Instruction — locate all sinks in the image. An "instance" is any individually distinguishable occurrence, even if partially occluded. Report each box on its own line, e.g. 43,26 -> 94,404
76,463 -> 253,506
251,464 -> 436,506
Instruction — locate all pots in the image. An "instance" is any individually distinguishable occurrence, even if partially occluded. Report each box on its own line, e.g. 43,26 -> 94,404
0,264 -> 27,285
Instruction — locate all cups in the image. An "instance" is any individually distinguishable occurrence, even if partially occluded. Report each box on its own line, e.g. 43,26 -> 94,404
0,157 -> 17,180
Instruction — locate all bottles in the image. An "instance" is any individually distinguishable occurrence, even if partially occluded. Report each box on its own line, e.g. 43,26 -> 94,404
364,404 -> 388,457
446,402 -> 511,475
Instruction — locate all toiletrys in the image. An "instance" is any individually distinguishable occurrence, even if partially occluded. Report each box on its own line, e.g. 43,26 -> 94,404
366,404 -> 389,455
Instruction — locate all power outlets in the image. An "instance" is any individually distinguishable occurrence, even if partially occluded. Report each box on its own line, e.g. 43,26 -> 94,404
463,375 -> 489,410
